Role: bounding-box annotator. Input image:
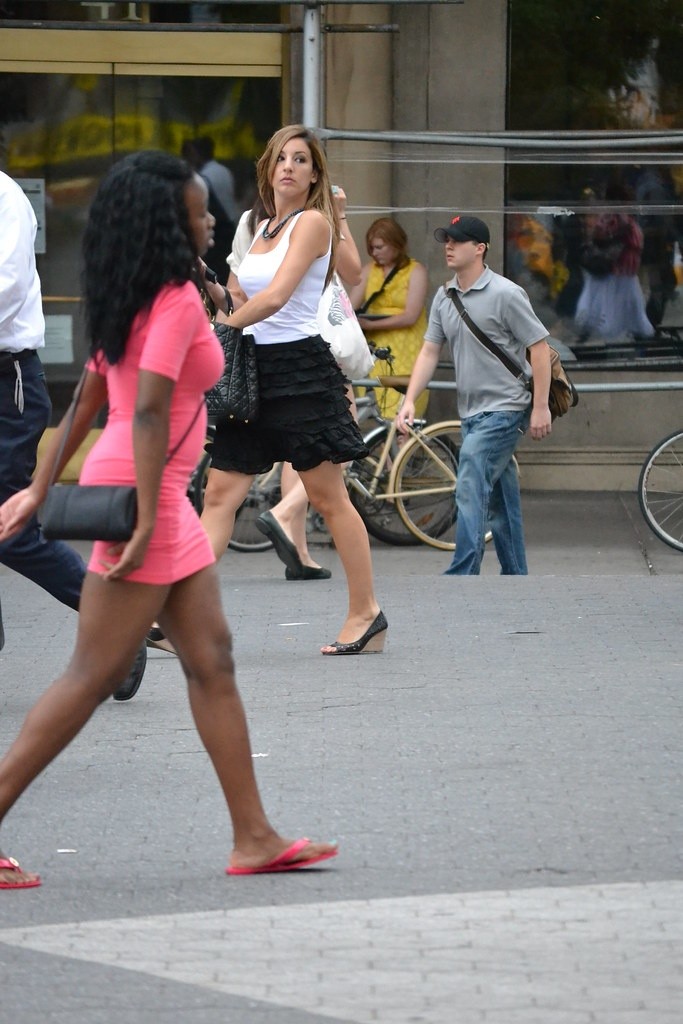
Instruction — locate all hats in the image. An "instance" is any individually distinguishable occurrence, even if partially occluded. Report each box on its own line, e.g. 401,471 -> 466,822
434,217 -> 491,247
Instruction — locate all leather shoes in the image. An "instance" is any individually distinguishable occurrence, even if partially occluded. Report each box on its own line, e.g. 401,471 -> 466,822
257,511 -> 302,577
285,561 -> 332,581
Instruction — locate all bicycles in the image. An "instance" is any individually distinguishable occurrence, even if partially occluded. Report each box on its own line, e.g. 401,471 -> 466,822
188,341 -> 524,555
636,425 -> 683,553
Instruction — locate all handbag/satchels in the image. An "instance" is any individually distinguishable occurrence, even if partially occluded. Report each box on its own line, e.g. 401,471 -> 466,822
319,274 -> 375,381
523,346 -> 577,425
42,483 -> 135,542
199,284 -> 258,426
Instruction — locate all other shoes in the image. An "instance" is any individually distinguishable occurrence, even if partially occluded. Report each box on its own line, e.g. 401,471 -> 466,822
112,639 -> 147,701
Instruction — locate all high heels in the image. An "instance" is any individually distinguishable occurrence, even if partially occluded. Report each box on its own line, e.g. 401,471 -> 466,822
322,611 -> 387,656
149,626 -> 166,642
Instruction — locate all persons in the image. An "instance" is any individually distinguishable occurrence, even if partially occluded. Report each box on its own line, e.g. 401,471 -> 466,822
395,217 -> 553,577
514,158 -> 683,346
252,186 -> 364,580
146,125 -> 389,657
0,150 -> 339,889
0,167 -> 149,701
187,135 -> 240,224
346,217 -> 429,512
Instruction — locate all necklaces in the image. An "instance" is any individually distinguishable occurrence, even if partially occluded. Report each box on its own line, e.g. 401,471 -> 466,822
262,208 -> 304,240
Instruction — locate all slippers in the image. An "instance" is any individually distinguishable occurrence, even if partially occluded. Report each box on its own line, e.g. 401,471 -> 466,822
224,837 -> 338,874
0,859 -> 42,887
